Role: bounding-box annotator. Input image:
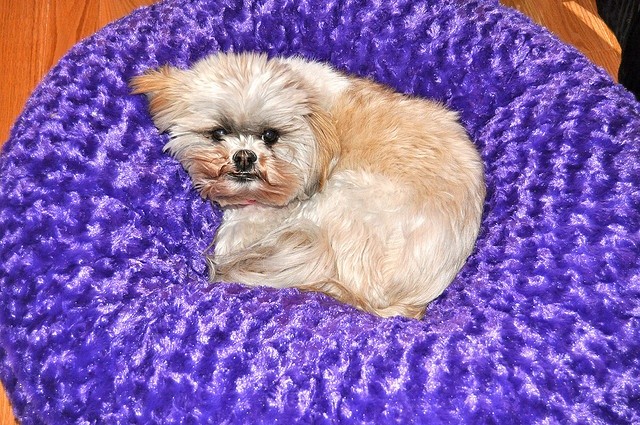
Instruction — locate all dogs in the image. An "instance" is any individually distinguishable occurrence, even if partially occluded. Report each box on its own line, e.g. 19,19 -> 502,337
129,49 -> 487,322
